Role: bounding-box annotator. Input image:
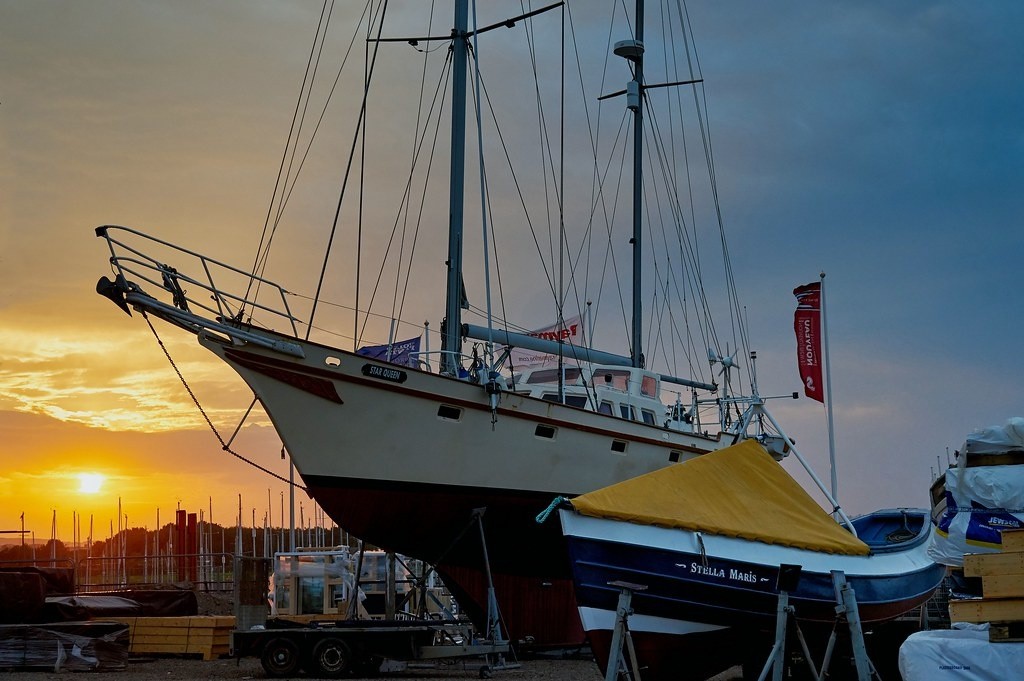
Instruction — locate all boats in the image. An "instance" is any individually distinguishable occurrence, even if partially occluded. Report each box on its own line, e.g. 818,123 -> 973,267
554,272 -> 949,681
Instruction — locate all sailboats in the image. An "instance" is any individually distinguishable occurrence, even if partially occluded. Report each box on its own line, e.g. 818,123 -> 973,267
94,0 -> 800,652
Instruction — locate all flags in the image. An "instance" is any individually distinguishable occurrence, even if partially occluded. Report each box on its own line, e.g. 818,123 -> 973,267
793,280 -> 825,402
493,313 -> 583,372
354,336 -> 422,370
